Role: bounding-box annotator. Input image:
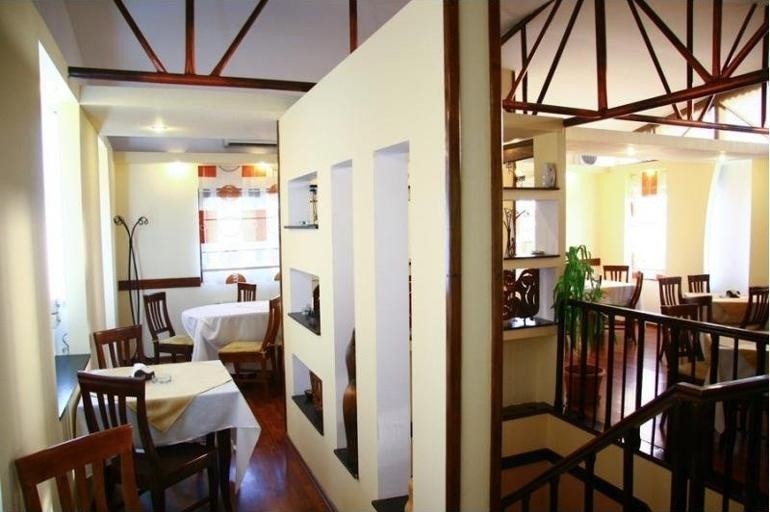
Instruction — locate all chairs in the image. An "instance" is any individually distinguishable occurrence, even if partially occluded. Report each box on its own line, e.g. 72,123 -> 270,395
142,290 -> 195,364
686,273 -> 712,295
218,296 -> 280,400
595,270 -> 644,346
236,281 -> 257,302
656,276 -> 684,360
680,295 -> 712,324
658,304 -> 713,429
737,284 -> 769,333
92,324 -> 149,369
10,422 -> 143,511
75,369 -> 221,509
602,264 -> 630,285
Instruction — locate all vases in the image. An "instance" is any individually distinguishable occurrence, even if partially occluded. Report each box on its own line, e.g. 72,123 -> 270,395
341,325 -> 357,472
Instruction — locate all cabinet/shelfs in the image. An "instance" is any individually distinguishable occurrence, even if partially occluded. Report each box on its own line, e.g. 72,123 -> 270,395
500,111 -> 565,409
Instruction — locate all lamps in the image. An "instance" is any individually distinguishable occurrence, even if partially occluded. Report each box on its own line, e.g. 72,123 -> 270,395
113,213 -> 153,363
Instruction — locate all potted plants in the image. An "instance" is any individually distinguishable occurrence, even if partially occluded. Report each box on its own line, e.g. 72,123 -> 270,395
551,246 -> 616,405
